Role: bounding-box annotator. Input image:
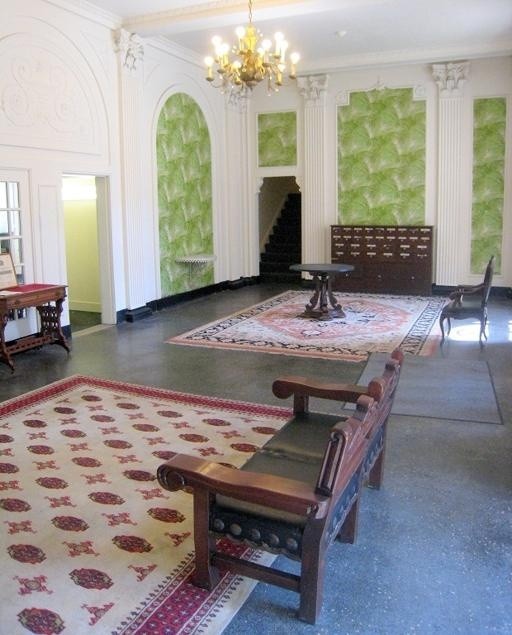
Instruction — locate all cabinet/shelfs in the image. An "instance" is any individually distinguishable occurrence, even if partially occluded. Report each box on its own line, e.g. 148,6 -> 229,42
329,224 -> 433,297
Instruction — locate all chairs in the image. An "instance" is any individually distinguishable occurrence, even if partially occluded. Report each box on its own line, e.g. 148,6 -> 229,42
439,255 -> 497,350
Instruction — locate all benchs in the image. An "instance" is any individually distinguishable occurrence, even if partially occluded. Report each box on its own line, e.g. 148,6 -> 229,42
156,349 -> 405,624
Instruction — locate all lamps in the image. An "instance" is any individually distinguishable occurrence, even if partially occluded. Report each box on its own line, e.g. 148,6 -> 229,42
205,2 -> 301,98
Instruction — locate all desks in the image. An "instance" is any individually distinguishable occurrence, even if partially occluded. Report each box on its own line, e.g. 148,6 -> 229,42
290,264 -> 355,321
0,284 -> 73,372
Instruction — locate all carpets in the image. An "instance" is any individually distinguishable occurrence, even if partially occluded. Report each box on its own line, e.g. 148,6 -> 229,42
162,289 -> 454,364
0,372 -> 354,634
